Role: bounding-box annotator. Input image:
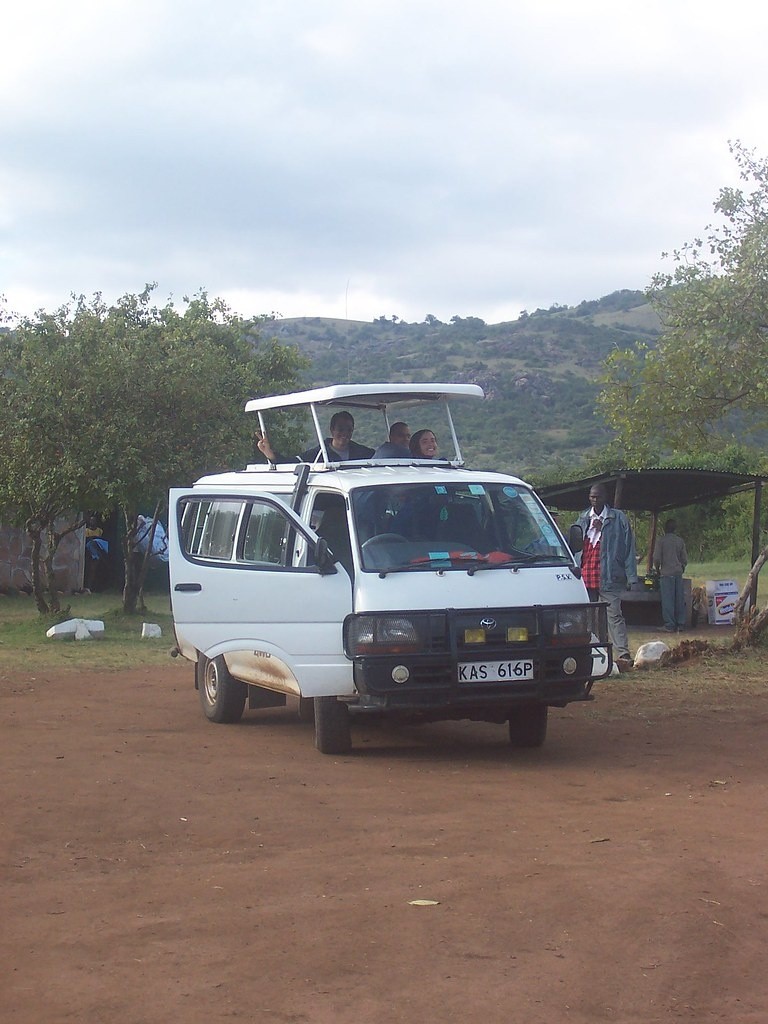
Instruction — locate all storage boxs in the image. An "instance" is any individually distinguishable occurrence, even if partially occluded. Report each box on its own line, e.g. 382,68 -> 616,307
706,578 -> 751,626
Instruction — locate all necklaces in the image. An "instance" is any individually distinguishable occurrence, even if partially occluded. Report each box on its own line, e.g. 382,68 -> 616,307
255,411 -> 375,510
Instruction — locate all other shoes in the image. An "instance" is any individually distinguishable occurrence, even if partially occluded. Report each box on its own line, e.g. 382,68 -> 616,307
657,626 -> 672,632
619,653 -> 631,661
676,626 -> 687,631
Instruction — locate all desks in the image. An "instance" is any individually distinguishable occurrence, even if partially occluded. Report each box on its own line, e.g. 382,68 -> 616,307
619,589 -> 666,627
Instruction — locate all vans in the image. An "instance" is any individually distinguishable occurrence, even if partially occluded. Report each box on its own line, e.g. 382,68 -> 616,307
166,382 -> 613,753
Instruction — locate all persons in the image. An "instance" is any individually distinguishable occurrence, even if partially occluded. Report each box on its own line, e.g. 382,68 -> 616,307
389,430 -> 437,538
653,519 -> 688,633
82,516 -> 103,592
576,482 -> 639,666
133,515 -> 169,595
526,510 -> 594,701
352,422 -> 411,539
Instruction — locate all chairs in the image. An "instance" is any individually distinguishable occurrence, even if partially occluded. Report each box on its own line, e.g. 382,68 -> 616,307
313,504 -> 352,569
411,503 -> 482,544
